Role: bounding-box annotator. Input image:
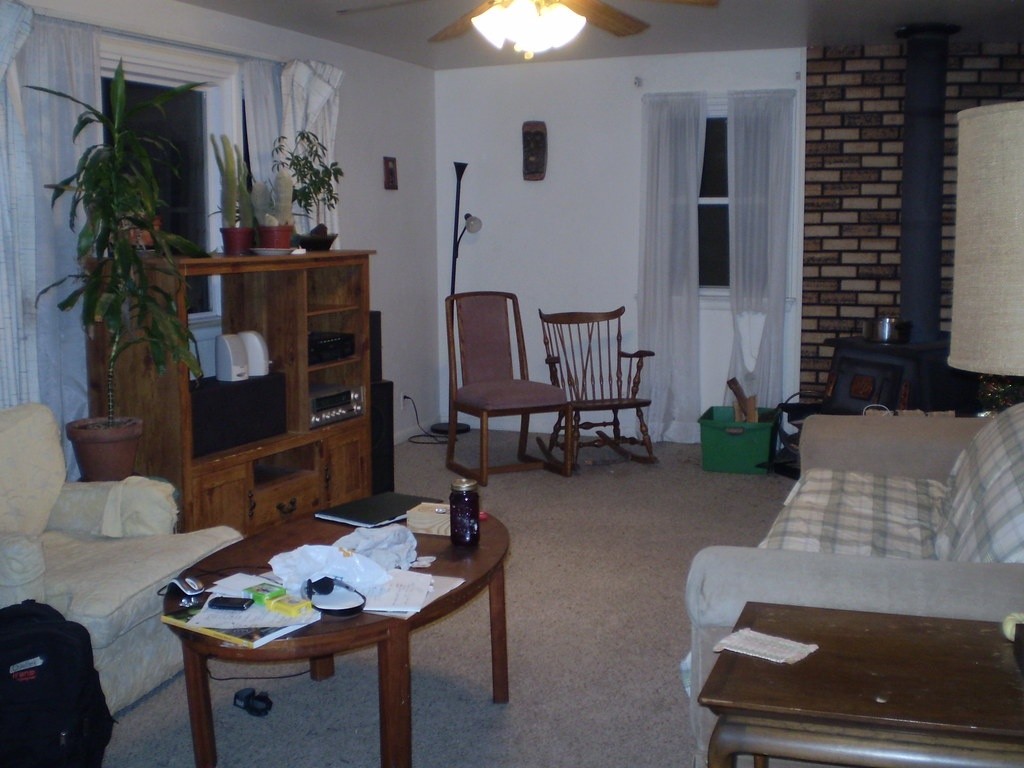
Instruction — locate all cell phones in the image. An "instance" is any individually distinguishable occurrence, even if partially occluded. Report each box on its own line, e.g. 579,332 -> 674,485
208,596 -> 254,611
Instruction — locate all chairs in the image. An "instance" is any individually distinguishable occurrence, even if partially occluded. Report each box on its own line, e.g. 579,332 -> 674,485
538,305 -> 660,477
445,286 -> 573,485
766,358 -> 904,479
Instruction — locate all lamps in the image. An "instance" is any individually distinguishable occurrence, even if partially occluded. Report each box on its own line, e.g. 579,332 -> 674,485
430,162 -> 483,436
469,0 -> 586,60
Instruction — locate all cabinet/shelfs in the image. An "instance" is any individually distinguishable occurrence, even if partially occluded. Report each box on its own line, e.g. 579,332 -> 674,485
85,248 -> 379,546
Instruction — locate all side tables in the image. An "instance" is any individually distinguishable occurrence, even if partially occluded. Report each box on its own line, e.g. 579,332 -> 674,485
697,599 -> 1024,768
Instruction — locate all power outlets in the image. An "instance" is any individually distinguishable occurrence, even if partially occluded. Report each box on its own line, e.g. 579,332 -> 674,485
401,392 -> 408,409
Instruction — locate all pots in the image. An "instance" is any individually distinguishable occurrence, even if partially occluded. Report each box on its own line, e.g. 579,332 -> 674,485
862,312 -> 913,344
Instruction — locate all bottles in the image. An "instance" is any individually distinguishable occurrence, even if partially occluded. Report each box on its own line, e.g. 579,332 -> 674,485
450,479 -> 480,546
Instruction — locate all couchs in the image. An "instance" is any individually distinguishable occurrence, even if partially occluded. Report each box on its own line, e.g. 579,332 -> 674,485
1,398 -> 244,718
685,401 -> 1024,768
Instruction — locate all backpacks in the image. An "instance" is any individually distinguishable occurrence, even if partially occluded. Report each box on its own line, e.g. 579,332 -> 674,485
0,600 -> 113,768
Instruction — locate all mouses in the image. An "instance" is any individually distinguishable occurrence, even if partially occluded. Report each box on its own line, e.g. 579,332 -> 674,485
167,577 -> 205,599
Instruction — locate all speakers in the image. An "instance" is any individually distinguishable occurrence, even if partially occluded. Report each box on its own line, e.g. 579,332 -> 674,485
238,331 -> 269,376
215,334 -> 249,382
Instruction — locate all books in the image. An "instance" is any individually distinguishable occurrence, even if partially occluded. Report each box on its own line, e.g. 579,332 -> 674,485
159,601 -> 323,649
313,491 -> 445,529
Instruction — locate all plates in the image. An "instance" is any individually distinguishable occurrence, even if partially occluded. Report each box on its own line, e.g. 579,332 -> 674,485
253,249 -> 295,256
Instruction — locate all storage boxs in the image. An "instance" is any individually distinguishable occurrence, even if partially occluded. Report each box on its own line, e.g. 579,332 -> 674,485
697,405 -> 781,471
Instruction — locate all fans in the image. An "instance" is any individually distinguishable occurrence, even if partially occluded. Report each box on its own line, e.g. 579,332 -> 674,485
427,0 -> 720,46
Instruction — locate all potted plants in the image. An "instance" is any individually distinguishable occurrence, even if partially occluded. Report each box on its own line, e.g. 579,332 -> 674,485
38,205 -> 204,481
252,173 -> 298,250
204,135 -> 254,256
21,63 -> 213,290
271,126 -> 338,251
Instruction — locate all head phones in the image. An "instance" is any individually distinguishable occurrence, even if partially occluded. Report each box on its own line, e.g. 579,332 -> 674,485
301,577 -> 366,617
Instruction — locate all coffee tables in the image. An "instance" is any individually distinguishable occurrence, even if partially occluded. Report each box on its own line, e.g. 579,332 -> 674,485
161,500 -> 512,768
